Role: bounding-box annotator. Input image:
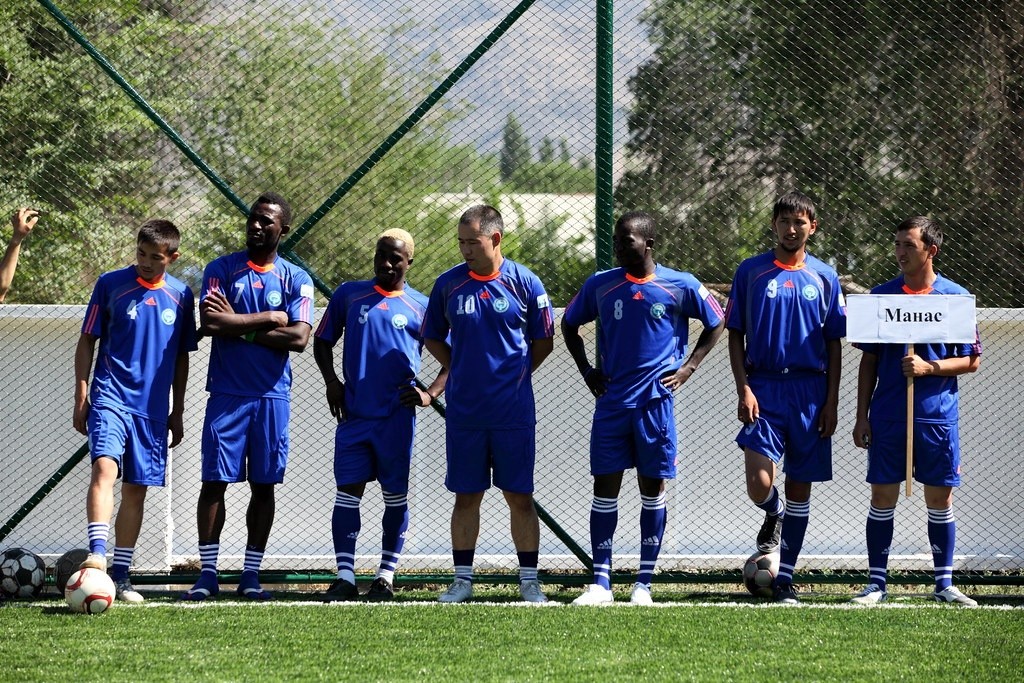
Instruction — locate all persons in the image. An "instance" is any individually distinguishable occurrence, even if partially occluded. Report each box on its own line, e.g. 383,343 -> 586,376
0,207 -> 38,303
725,193 -> 846,602
73,220 -> 198,600
181,194 -> 313,600
313,229 -> 451,596
421,206 -> 554,601
561,212 -> 725,604
851,216 -> 983,606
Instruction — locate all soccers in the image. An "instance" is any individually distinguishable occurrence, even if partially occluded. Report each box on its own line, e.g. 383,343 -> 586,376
52,549 -> 92,595
0,547 -> 47,602
65,568 -> 117,614
743,551 -> 780,597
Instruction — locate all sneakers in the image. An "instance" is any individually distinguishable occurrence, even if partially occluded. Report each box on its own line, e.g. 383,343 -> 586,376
80,554 -> 108,572
852,584 -> 888,605
521,580 -> 548,603
933,585 -> 977,607
756,498 -> 785,553
116,579 -> 144,603
631,583 -> 654,607
237,583 -> 272,602
776,579 -> 800,605
437,581 -> 473,603
366,578 -> 394,601
179,588 -> 219,602
318,578 -> 360,603
572,584 -> 614,607
